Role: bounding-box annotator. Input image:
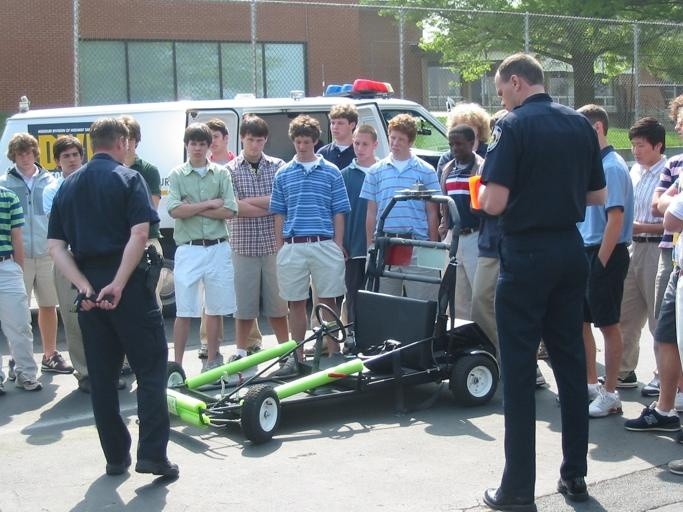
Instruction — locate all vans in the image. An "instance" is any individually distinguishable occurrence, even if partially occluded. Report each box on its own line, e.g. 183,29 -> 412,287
1,78 -> 448,258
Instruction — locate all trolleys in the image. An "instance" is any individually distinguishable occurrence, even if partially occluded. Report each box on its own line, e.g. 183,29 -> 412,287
168,188 -> 500,443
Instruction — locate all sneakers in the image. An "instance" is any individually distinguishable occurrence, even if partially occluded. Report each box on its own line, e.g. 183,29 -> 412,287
198,342 -> 329,373
536,343 -> 683,474
0,351 -> 130,396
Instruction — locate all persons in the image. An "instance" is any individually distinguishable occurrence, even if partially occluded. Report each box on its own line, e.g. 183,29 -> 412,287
167,102 -> 443,384
476,52 -> 607,510
623,95 -> 683,476
440,100 -> 669,417
0,114 -> 161,393
45,118 -> 179,479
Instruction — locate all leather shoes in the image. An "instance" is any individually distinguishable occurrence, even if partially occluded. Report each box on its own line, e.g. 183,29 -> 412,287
136,458 -> 178,476
483,488 -> 537,512
106,452 -> 131,475
558,476 -> 588,500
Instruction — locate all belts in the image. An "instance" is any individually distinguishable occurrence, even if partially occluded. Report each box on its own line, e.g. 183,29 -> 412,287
0,255 -> 10,261
632,236 -> 662,242
285,236 -> 328,243
383,232 -> 411,238
185,239 -> 224,245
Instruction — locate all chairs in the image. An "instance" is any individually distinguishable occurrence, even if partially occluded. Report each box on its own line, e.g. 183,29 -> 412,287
299,290 -> 437,384
